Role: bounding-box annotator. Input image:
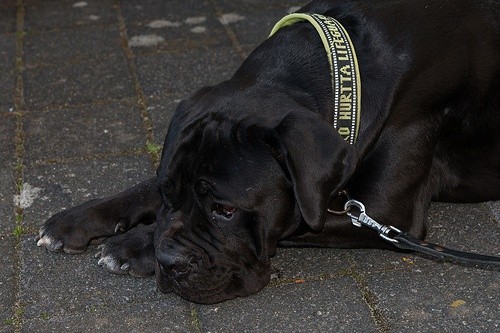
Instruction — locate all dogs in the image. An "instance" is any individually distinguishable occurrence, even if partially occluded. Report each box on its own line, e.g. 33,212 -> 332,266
34,0 -> 500,305
